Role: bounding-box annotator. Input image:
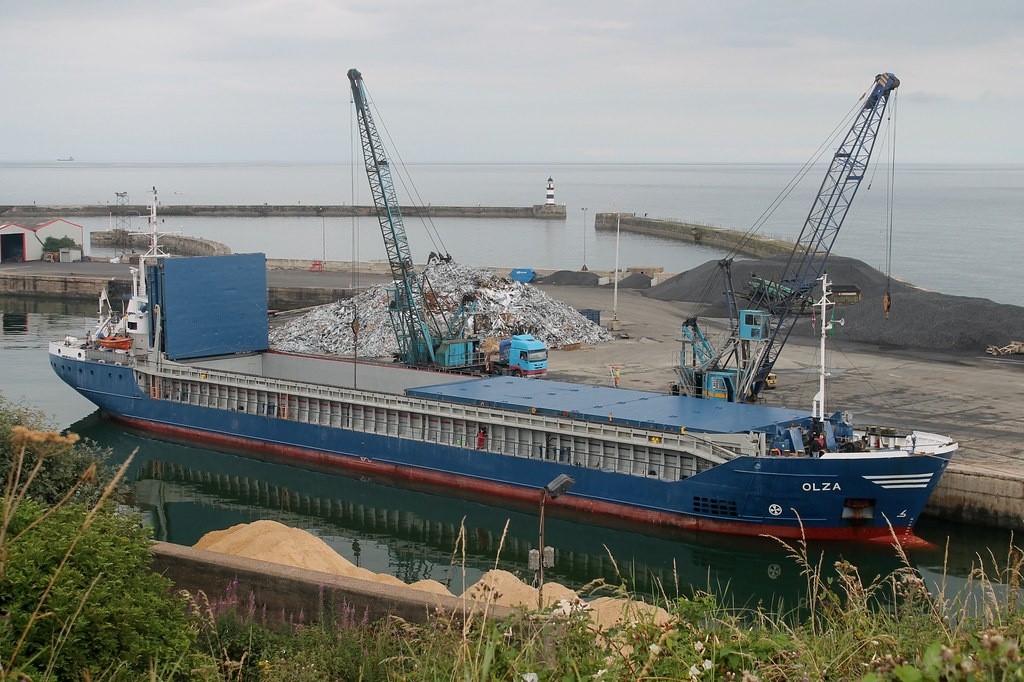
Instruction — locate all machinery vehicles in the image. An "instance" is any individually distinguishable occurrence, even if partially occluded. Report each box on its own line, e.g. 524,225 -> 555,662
347,68 -> 550,378
664,71 -> 901,402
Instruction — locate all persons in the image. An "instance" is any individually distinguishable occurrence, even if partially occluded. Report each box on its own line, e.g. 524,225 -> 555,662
476,426 -> 487,450
810,434 -> 825,458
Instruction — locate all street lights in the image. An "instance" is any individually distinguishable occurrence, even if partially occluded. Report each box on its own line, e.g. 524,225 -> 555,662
582,208 -> 587,266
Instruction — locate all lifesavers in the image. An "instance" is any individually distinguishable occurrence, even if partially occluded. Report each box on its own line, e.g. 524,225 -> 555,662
774,448 -> 781,456
819,437 -> 824,447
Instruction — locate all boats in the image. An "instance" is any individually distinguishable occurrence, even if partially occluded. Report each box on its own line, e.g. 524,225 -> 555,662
57,156 -> 72,162
46,188 -> 961,543
